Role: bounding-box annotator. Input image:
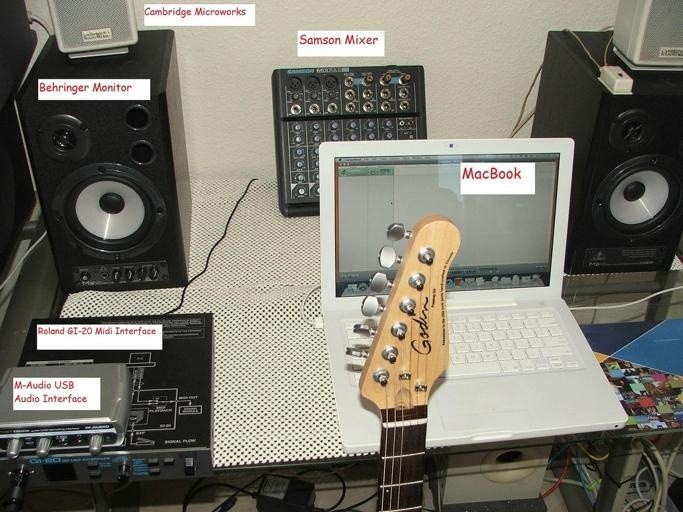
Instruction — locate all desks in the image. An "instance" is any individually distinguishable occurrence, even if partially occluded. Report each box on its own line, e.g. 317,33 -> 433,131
1,0 -> 683,512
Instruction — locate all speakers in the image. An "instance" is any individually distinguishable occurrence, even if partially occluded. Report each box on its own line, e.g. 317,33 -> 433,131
530,31 -> 683,275
47,0 -> 139,59
434,445 -> 553,505
14,29 -> 192,294
612,0 -> 683,71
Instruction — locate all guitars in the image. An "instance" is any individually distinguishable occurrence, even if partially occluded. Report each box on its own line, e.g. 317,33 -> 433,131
346,214 -> 461,511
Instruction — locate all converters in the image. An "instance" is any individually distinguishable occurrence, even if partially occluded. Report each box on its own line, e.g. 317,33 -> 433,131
255,474 -> 316,512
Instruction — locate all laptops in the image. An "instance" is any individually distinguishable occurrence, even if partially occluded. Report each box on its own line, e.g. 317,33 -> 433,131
317,137 -> 629,454
